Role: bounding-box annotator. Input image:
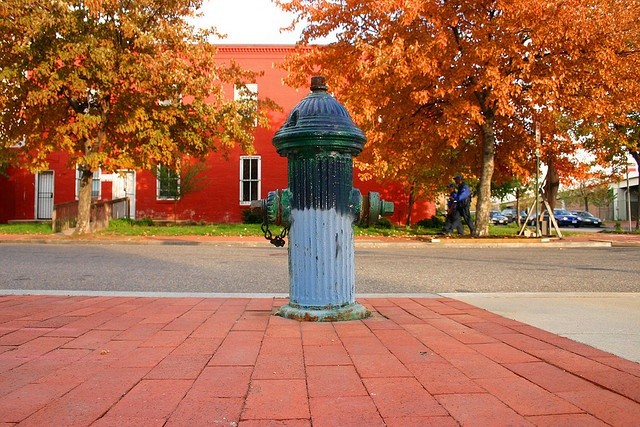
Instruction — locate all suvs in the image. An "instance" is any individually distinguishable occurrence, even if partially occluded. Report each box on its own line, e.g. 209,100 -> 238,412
543,209 -> 582,228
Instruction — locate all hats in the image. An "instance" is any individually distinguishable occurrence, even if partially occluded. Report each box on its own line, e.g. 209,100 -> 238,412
453,175 -> 465,180
447,184 -> 455,188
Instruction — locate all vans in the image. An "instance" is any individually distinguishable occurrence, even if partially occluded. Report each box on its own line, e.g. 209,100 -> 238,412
502,209 -> 527,223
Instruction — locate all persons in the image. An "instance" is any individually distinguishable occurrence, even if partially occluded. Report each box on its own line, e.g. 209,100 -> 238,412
446,184 -> 464,235
436,175 -> 476,237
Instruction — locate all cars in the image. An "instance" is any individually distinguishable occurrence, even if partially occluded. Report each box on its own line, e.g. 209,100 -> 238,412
489,211 -> 508,225
569,210 -> 602,227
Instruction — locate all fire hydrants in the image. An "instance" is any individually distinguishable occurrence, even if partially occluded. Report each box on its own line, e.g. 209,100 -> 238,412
250,76 -> 394,322
